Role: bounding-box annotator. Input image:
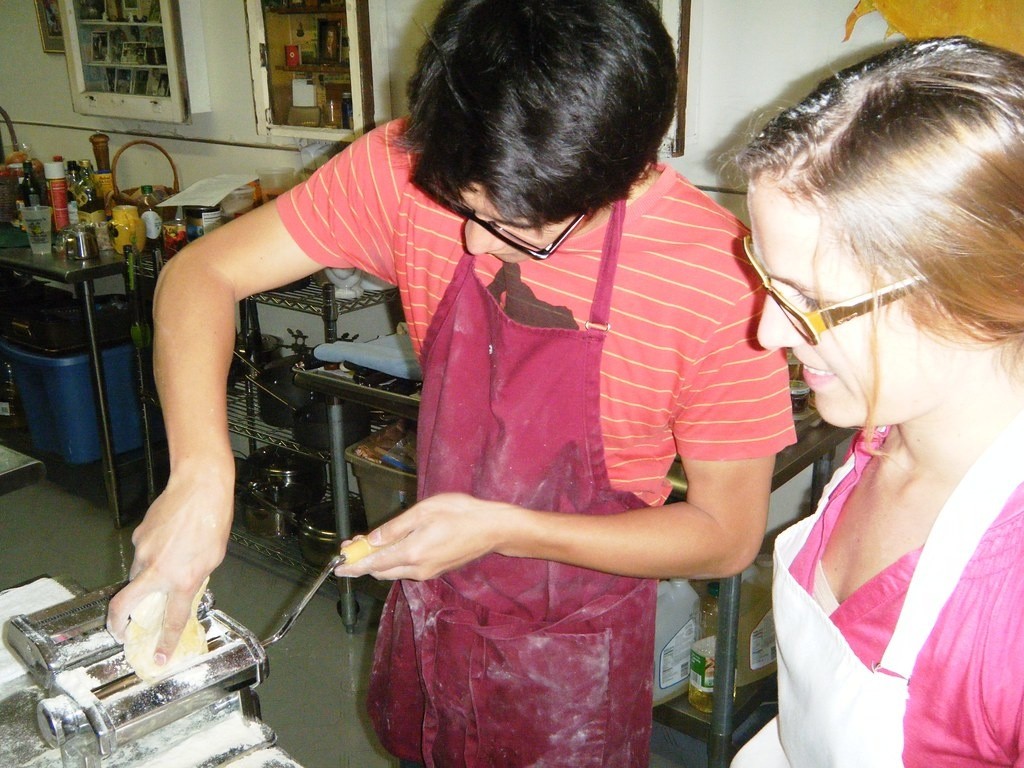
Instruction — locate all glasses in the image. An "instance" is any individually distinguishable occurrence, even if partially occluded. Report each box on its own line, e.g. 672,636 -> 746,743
411,149 -> 595,261
742,232 -> 931,346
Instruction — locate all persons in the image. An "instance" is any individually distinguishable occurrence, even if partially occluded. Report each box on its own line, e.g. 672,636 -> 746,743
104,0 -> 797,768
722,36 -> 1024,768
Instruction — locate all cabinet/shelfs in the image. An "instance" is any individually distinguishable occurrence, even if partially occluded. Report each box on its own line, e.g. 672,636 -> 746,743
124,244 -> 402,589
293,356 -> 853,768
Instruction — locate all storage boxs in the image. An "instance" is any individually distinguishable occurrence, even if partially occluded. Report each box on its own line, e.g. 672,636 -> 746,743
0,293 -> 163,471
343,418 -> 420,532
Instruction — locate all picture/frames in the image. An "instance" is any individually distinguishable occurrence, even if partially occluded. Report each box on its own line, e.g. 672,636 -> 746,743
34,0 -> 65,53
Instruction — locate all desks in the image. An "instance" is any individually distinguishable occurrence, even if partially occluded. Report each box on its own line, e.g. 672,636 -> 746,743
0,236 -> 165,527
